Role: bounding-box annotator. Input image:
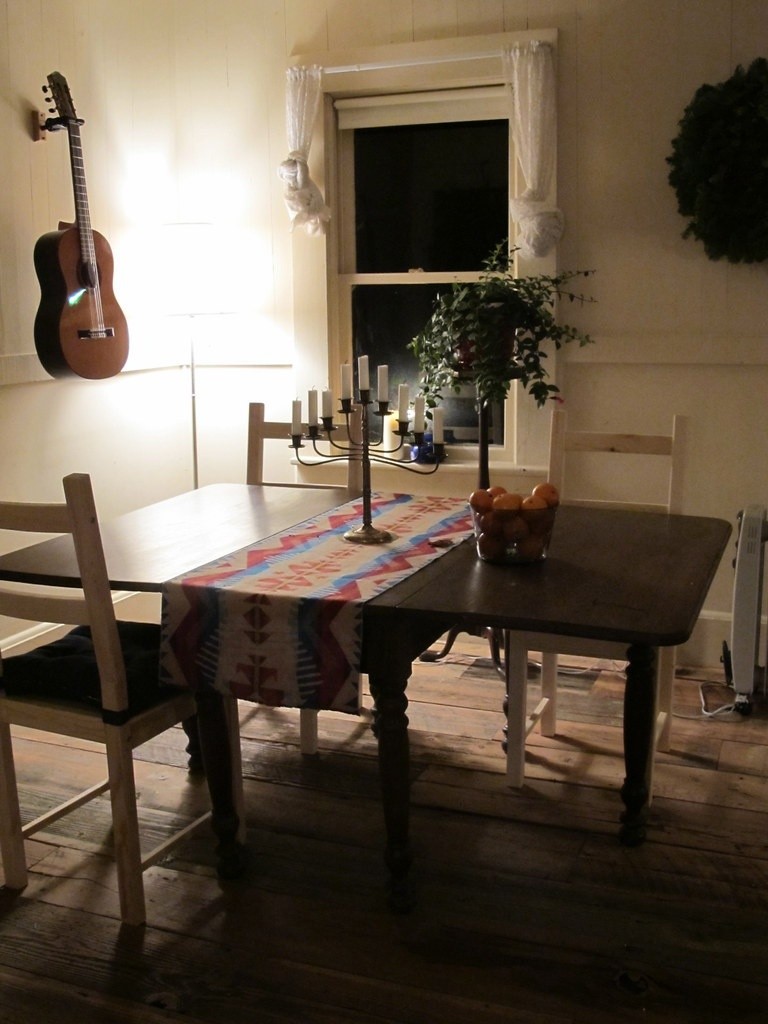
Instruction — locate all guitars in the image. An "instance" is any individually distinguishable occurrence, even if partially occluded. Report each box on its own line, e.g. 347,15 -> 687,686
33,71 -> 130,381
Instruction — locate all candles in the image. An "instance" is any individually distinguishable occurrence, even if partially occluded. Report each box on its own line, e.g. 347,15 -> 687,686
290,396 -> 302,435
432,403 -> 443,443
383,400 -> 402,460
377,363 -> 388,401
414,392 -> 424,433
340,360 -> 350,399
321,386 -> 332,417
357,353 -> 369,389
398,378 -> 408,421
308,385 -> 318,425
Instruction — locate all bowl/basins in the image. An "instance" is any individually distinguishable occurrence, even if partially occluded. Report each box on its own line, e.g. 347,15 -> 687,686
468,502 -> 560,564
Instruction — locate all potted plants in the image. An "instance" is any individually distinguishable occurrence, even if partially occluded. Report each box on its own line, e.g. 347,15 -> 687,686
411,240 -> 596,434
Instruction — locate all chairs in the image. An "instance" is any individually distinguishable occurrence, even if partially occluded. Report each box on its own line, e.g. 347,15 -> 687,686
244,400 -> 364,753
0,471 -> 247,925
507,411 -> 692,807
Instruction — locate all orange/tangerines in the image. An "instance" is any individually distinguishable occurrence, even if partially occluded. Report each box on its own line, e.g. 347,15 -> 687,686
468,483 -> 559,562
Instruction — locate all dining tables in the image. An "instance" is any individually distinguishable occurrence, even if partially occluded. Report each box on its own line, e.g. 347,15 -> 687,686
0,484 -> 730,853
